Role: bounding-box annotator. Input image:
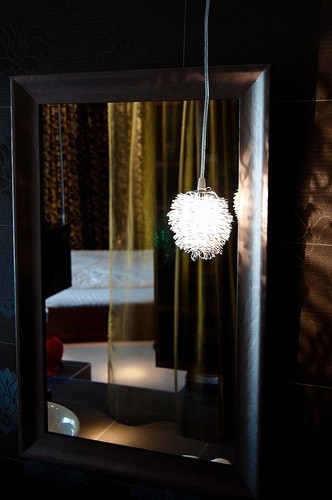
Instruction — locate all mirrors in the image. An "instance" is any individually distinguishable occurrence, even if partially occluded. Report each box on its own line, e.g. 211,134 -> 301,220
9,60 -> 271,497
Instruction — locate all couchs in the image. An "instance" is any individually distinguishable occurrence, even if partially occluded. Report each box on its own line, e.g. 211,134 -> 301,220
44,249 -> 155,340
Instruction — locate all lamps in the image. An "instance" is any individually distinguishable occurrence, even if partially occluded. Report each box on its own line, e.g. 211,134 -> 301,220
166,0 -> 233,261
